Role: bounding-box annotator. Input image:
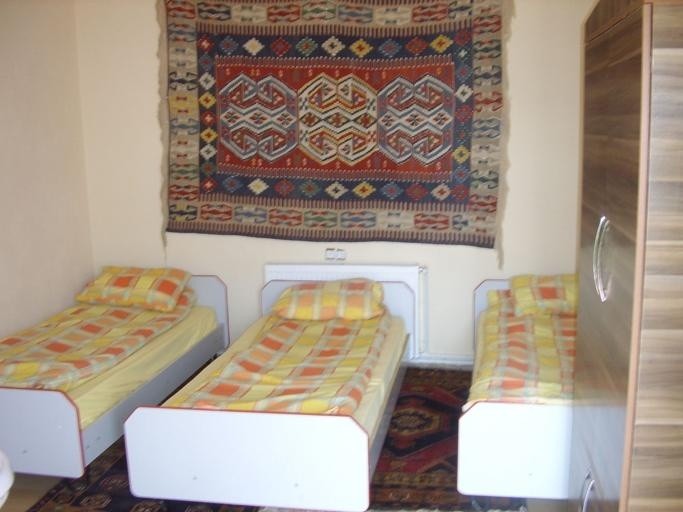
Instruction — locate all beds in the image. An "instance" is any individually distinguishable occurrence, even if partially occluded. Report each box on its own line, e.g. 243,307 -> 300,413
116,279 -> 415,512
2,267 -> 229,479
457,277 -> 576,499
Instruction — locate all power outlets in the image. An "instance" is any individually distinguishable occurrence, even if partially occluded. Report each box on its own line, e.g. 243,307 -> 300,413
336,249 -> 345,259
325,249 -> 334,259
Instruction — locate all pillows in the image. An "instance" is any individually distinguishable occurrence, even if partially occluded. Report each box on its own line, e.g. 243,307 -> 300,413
71,265 -> 190,311
275,281 -> 381,321
510,274 -> 576,315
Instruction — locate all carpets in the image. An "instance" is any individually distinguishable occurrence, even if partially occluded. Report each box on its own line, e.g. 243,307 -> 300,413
28,356 -> 529,507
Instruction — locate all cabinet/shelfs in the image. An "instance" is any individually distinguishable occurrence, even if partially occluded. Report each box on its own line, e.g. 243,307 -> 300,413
570,0 -> 683,511
